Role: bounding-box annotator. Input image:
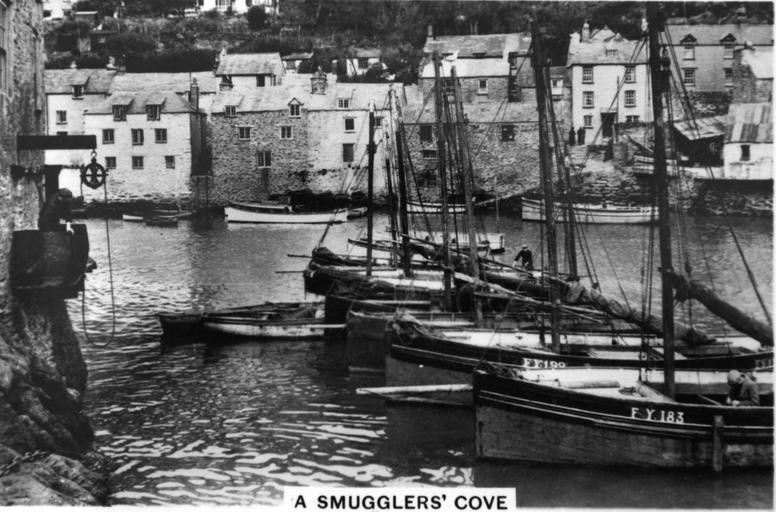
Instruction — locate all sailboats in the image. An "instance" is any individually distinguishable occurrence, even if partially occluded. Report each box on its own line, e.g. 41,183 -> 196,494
384,2 -> 770,408
347,3 -> 664,387
301,82 -> 507,293
473,3 -> 774,475
323,27 -> 598,339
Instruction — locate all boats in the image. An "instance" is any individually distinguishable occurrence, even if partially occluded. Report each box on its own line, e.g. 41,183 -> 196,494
89,196 -> 196,223
140,210 -> 178,226
227,197 -> 305,214
158,299 -> 336,341
331,206 -> 369,217
396,193 -> 477,215
348,226 -> 507,255
224,205 -> 347,223
521,197 -> 660,224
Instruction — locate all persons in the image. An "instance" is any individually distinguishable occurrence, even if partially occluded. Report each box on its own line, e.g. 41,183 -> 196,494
567,125 -> 587,146
452,282 -> 475,314
38,188 -> 76,235
513,244 -> 532,270
725,368 -> 762,407
